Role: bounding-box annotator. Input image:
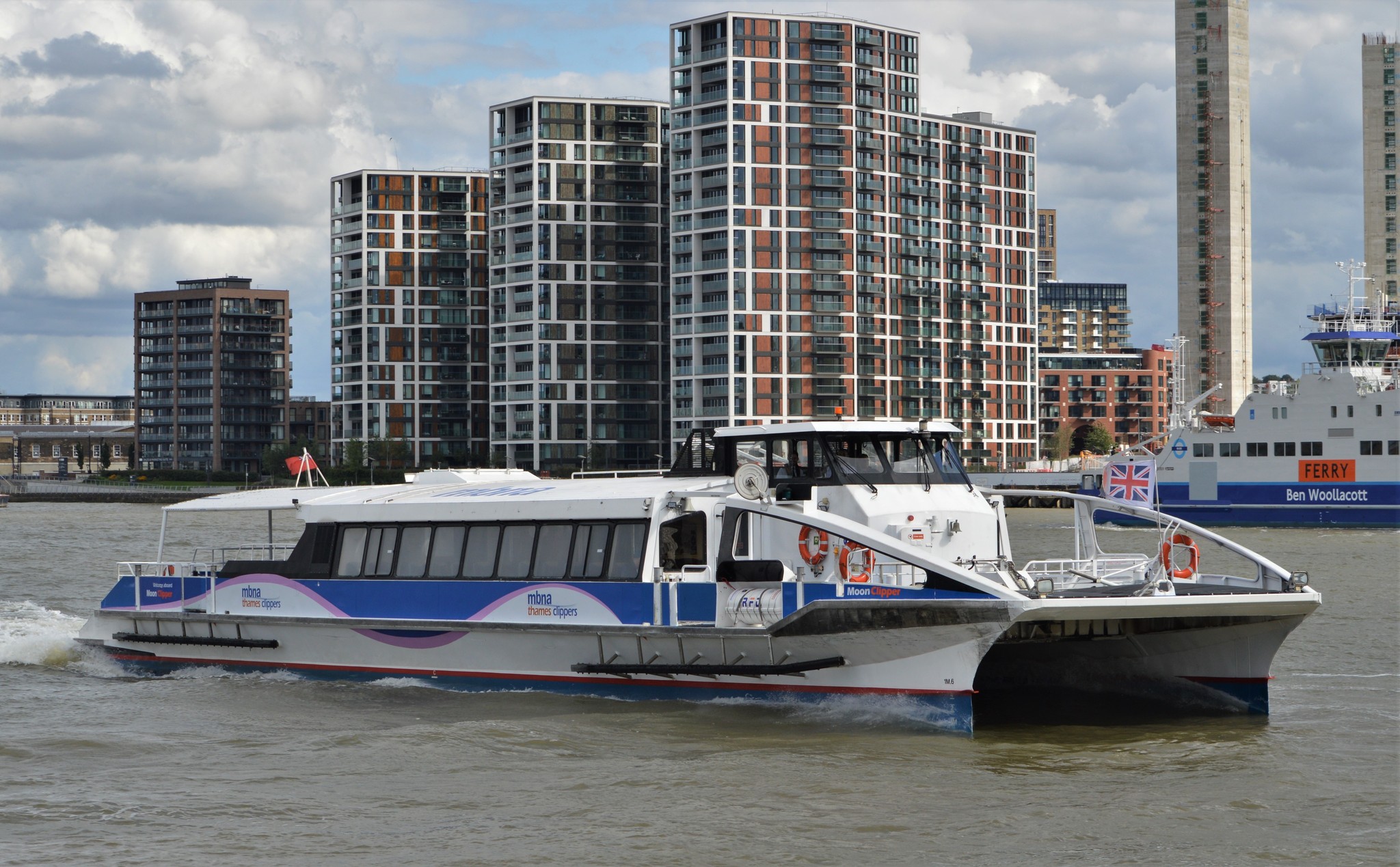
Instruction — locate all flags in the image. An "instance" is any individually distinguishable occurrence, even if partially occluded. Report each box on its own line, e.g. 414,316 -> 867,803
287,454 -> 318,475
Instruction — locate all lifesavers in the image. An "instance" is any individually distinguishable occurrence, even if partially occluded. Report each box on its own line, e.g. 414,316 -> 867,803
1160,534 -> 1200,578
798,526 -> 828,566
839,541 -> 875,583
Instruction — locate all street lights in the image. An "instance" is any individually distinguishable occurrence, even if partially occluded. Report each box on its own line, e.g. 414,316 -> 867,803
244,463 -> 249,486
1134,413 -> 1140,453
367,457 -> 376,486
1156,414 -> 1160,448
577,455 -> 586,479
654,454 -> 664,470
1058,416 -> 1064,471
996,450 -> 1004,473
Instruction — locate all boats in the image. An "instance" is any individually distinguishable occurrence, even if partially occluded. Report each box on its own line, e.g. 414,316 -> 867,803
1202,415 -> 1234,427
67,406 -> 1322,742
1067,258 -> 1400,535
